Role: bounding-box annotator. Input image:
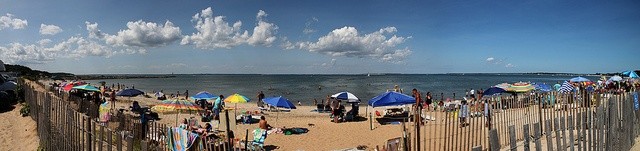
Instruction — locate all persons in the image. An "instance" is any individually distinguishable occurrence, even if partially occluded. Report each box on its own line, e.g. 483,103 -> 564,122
321,96 -> 325,104
331,101 -> 359,121
484,99 -> 493,126
131,101 -> 161,121
70,91 -> 106,105
158,89 -> 166,100
298,100 -> 302,106
313,98 -> 318,106
260,116 -> 273,130
553,81 -> 561,91
592,87 -> 625,95
178,118 -> 187,129
466,89 -> 469,98
412,88 -> 423,126
385,84 -> 405,94
433,92 -> 459,111
459,99 -> 469,127
110,90 -> 116,108
192,123 -> 211,134
426,91 -> 432,110
326,95 -> 330,105
184,89 -> 188,99
257,90 -> 265,107
117,83 -> 120,91
469,87 -> 475,98
176,90 -> 180,97
318,84 -> 321,90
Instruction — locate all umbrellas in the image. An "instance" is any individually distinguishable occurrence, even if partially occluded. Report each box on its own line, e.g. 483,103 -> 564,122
571,75 -> 591,82
507,81 -> 532,92
151,96 -> 206,127
621,69 -> 639,78
190,92 -> 219,100
99,81 -> 106,83
262,96 -> 299,126
482,86 -> 504,99
72,83 -> 103,92
330,91 -> 361,103
606,74 -> 623,87
533,81 -> 552,92
495,82 -> 511,90
114,88 -> 144,111
73,81 -> 87,86
64,82 -> 73,90
224,93 -> 250,131
527,80 -> 540,89
